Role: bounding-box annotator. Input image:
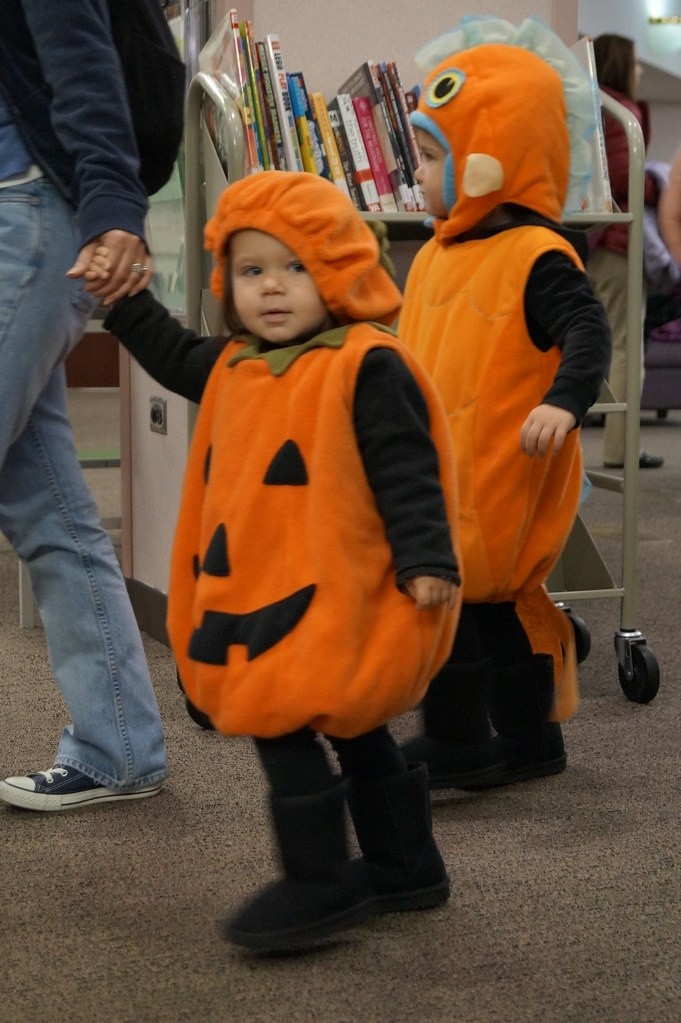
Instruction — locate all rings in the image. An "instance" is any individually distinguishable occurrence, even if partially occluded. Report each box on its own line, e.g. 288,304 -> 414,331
132,263 -> 147,274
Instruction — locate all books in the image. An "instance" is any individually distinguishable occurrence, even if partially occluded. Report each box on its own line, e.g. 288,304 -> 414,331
572,35 -> 612,212
198,8 -> 424,213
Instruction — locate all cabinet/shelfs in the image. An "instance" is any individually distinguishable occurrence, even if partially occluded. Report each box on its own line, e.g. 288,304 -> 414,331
119,0 -> 662,733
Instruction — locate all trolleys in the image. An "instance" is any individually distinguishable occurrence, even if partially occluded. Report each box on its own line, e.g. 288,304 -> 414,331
182,71 -> 661,731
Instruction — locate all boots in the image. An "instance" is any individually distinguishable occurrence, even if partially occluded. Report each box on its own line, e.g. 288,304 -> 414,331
226,775 -> 364,950
483,653 -> 568,778
396,665 -> 525,787
326,750 -> 449,927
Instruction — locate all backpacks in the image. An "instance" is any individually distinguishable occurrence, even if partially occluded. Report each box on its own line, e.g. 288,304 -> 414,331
0,0 -> 185,195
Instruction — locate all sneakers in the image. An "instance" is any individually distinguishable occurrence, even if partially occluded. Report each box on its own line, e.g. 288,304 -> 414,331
0,764 -> 162,813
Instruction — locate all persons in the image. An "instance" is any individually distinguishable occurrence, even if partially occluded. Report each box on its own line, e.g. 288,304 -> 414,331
83,172 -> 463,952
398,48 -> 611,801
0,0 -> 185,810
589,32 -> 681,469
